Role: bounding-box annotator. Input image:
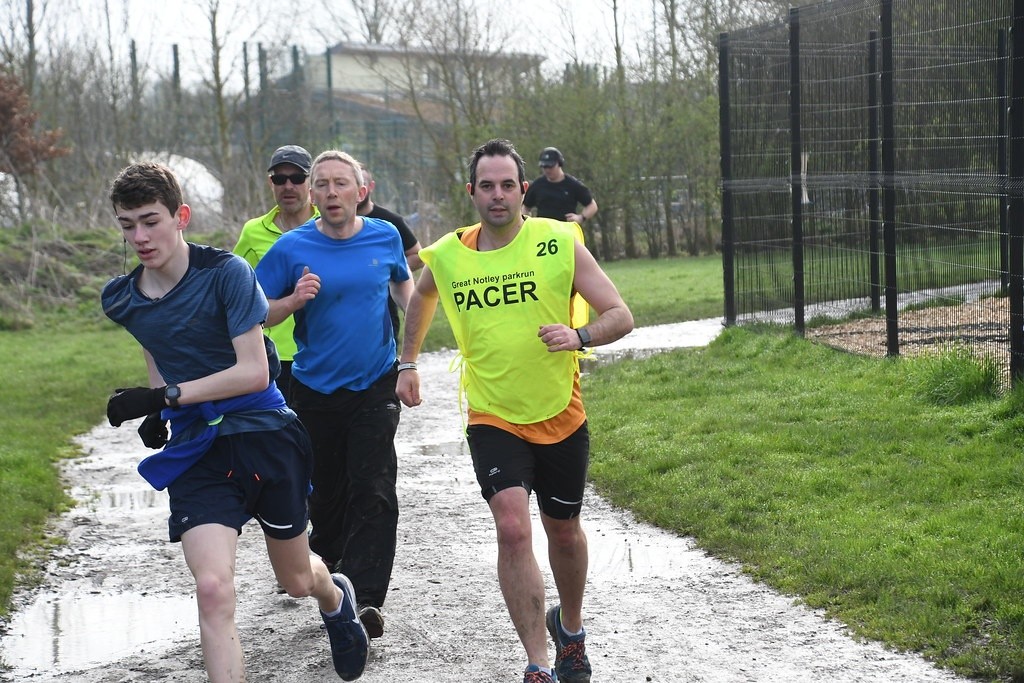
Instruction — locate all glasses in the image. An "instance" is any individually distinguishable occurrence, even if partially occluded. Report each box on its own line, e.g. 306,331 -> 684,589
541,163 -> 556,168
271,173 -> 309,185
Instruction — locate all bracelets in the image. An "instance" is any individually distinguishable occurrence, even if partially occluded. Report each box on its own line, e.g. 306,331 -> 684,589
582,214 -> 586,222
397,362 -> 417,371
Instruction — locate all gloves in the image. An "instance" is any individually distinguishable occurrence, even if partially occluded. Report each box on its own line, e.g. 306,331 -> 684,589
138,410 -> 169,449
107,386 -> 170,427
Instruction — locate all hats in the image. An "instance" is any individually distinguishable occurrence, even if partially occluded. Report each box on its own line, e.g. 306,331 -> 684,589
538,146 -> 565,166
268,144 -> 313,173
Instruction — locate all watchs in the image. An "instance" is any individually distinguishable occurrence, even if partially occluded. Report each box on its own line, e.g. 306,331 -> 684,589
574,328 -> 591,351
165,383 -> 181,409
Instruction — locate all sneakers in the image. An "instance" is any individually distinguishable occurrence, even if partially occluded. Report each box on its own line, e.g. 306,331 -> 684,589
356,601 -> 384,638
545,603 -> 592,683
319,573 -> 371,683
523,663 -> 558,683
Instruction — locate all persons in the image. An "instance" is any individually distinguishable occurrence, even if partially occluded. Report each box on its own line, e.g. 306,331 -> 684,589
256,150 -> 416,636
396,139 -> 635,683
100,164 -> 370,683
231,145 -> 320,594
357,167 -> 422,348
522,147 -> 599,225
